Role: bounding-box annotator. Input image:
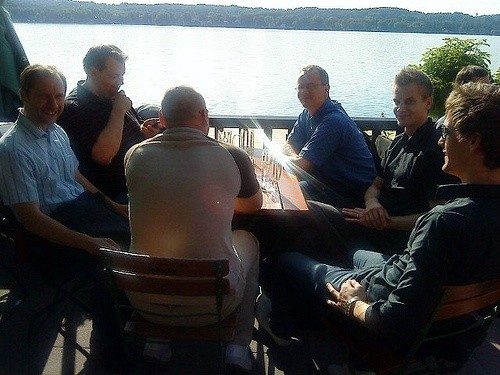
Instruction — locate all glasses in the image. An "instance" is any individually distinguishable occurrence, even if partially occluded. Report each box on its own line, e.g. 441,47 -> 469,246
295,84 -> 320,92
440,125 -> 452,140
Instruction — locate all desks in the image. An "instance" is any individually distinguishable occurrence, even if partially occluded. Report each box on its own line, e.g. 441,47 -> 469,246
232,158 -> 312,219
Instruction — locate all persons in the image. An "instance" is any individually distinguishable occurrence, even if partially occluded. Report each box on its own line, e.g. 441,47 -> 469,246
0,65 -> 145,361
285,63 -> 376,209
435,65 -> 490,129
304,67 -> 459,260
56,43 -> 160,205
124,85 -> 262,367
254,79 -> 500,375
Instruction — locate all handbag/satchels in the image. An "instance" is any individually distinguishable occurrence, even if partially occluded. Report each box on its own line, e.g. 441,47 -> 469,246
362,131 -> 383,176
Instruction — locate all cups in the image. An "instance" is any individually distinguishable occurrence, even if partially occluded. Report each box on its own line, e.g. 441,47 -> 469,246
216,129 -> 232,144
261,150 -> 283,193
232,127 -> 254,160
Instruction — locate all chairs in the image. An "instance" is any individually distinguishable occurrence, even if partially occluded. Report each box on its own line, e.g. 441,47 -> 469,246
0,199 -> 99,363
99,247 -> 235,375
373,135 -> 394,168
319,267 -> 500,375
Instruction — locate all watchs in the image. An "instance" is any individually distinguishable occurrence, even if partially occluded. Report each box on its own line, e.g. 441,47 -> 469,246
346,299 -> 358,320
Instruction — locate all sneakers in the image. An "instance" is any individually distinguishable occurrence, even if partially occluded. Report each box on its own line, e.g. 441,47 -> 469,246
255,294 -> 291,347
225,344 -> 255,372
143,337 -> 173,363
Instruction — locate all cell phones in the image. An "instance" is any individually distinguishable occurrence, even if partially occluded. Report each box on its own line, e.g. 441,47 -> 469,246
139,123 -> 166,140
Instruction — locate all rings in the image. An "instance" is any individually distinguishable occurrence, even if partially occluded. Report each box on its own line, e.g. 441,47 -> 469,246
357,214 -> 358,218
